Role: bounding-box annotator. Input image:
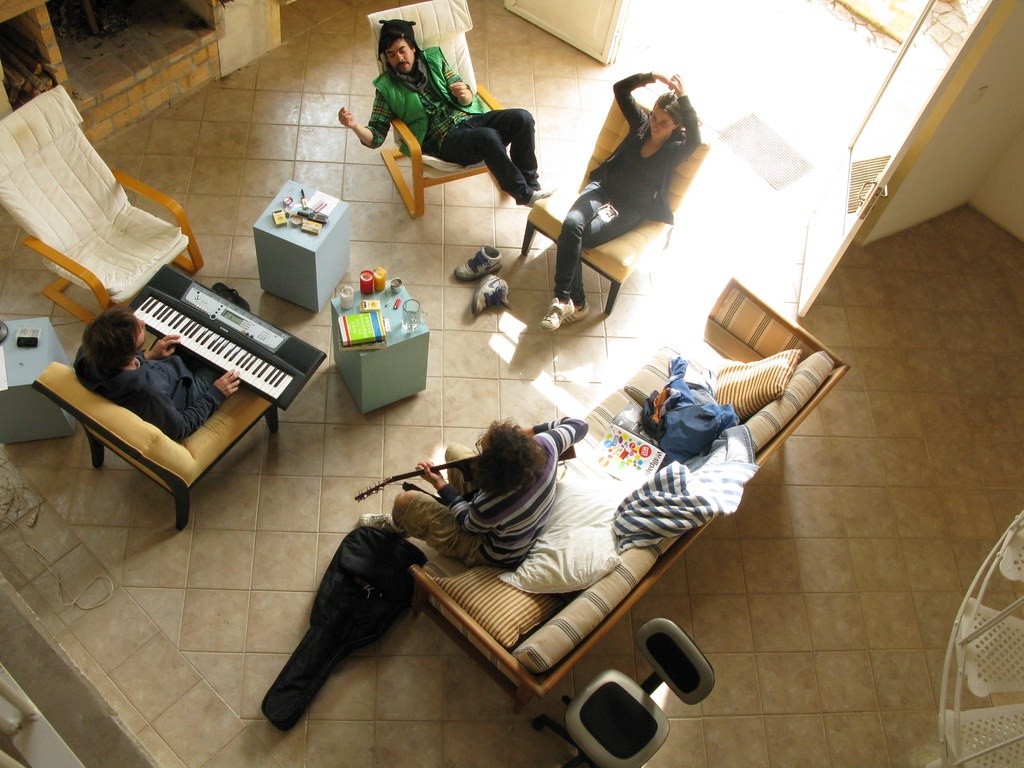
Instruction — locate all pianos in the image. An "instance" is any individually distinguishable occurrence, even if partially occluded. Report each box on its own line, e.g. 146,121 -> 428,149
125,263 -> 327,411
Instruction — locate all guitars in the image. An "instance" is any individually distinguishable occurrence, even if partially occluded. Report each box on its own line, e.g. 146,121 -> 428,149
352,444 -> 578,503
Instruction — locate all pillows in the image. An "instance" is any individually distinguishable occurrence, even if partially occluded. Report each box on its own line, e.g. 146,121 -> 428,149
432,565 -> 559,648
497,476 -> 628,593
715,347 -> 802,423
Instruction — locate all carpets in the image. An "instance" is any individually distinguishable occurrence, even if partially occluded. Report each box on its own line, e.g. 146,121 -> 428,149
718,113 -> 813,190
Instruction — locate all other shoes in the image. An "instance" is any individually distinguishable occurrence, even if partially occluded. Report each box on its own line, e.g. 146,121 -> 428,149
212,282 -> 250,311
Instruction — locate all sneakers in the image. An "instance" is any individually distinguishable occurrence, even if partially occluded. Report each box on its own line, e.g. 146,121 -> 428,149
453,244 -> 503,280
539,297 -> 575,332
560,299 -> 589,325
471,274 -> 509,315
359,514 -> 411,538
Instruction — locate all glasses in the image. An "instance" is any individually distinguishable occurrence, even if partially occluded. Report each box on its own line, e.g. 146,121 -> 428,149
475,438 -> 483,456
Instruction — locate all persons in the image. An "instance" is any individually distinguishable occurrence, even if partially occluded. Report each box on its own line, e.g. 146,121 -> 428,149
338,19 -> 556,206
359,416 -> 588,570
539,71 -> 701,332
73,303 -> 240,443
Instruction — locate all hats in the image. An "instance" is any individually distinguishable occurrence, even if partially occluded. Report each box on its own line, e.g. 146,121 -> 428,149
378,19 -> 421,61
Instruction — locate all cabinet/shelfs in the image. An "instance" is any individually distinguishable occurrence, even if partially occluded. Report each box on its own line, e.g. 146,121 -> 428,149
330,277 -> 430,414
0,317 -> 74,445
252,180 -> 351,312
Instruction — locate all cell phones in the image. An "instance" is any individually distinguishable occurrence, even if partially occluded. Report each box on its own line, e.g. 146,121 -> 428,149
308,213 -> 329,223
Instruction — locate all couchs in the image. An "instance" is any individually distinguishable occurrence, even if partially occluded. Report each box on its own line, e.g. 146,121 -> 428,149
408,277 -> 851,712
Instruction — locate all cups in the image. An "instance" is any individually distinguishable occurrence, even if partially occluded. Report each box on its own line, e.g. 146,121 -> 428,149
374,268 -> 386,291
390,279 -> 402,293
340,286 -> 353,310
360,270 -> 374,294
402,299 -> 429,333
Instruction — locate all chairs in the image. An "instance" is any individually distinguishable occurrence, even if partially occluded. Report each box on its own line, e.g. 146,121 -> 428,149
522,96 -> 709,316
532,618 -> 714,768
31,336 -> 279,531
367,0 -> 509,218
1,85 -> 204,323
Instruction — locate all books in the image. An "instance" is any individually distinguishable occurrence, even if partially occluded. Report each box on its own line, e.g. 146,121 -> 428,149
337,310 -> 385,351
